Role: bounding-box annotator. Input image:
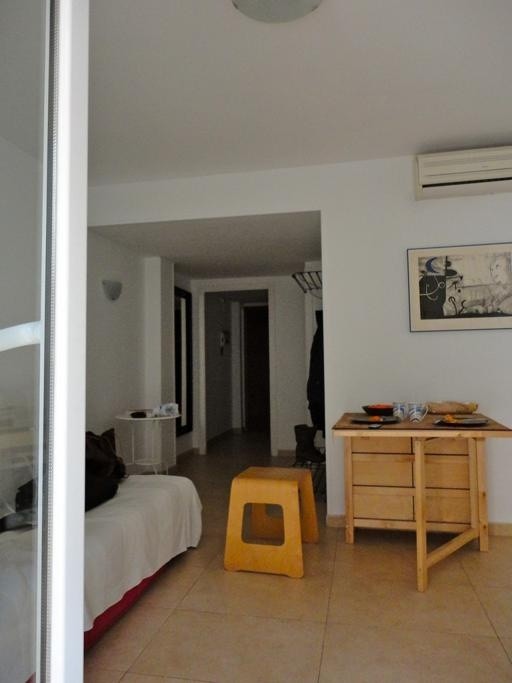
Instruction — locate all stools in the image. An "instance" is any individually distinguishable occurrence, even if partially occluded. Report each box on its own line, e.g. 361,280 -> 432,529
224,467 -> 319,579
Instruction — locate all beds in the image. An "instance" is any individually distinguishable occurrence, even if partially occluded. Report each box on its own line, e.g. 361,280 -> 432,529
0,475 -> 204,683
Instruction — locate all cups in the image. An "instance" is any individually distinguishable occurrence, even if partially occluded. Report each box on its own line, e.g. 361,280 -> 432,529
392,400 -> 409,421
407,400 -> 429,424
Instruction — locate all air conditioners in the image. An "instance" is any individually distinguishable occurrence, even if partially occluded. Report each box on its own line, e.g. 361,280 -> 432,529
412,143 -> 512,200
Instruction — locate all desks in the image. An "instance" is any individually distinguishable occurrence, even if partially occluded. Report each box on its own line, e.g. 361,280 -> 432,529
335,413 -> 512,591
116,414 -> 180,475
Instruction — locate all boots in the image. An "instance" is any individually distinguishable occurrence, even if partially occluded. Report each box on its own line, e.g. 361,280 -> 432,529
294,423 -> 325,462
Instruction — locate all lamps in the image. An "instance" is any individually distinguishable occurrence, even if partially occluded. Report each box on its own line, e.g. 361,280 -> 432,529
232,0 -> 321,24
103,280 -> 123,302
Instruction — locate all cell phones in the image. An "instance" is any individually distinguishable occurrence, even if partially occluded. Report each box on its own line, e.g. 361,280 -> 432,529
368,423 -> 381,429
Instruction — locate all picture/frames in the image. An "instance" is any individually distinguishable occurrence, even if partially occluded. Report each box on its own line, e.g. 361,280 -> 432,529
405,241 -> 512,332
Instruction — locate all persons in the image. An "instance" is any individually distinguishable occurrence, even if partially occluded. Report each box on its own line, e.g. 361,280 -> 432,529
0,426 -> 131,535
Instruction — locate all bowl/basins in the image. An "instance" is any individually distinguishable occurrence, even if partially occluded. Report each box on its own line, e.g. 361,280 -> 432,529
362,405 -> 393,415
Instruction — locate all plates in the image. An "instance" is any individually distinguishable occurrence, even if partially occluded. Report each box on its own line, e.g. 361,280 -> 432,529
351,415 -> 400,424
431,415 -> 488,427
